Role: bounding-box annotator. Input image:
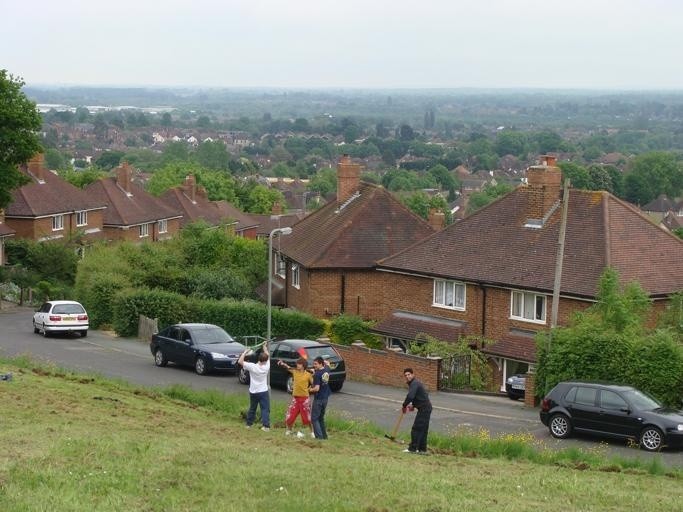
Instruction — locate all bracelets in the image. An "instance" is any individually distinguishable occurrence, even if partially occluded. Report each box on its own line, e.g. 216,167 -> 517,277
243,350 -> 247,355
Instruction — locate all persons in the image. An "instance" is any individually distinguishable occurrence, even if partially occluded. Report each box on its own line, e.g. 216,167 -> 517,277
276,358 -> 314,437
401,367 -> 431,453
308,356 -> 330,440
237,342 -> 270,431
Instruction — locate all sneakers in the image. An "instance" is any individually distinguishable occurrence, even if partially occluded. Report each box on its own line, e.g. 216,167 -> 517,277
244,424 -> 328,441
401,447 -> 429,454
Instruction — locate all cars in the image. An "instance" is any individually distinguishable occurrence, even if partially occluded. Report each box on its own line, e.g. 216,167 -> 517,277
150,323 -> 345,392
505,373 -> 525,400
32,300 -> 89,337
540,382 -> 683,452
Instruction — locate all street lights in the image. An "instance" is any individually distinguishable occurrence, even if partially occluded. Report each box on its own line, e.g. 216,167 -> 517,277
266,227 -> 292,399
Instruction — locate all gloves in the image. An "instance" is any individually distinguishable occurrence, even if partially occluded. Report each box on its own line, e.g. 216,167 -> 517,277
402,404 -> 414,414
